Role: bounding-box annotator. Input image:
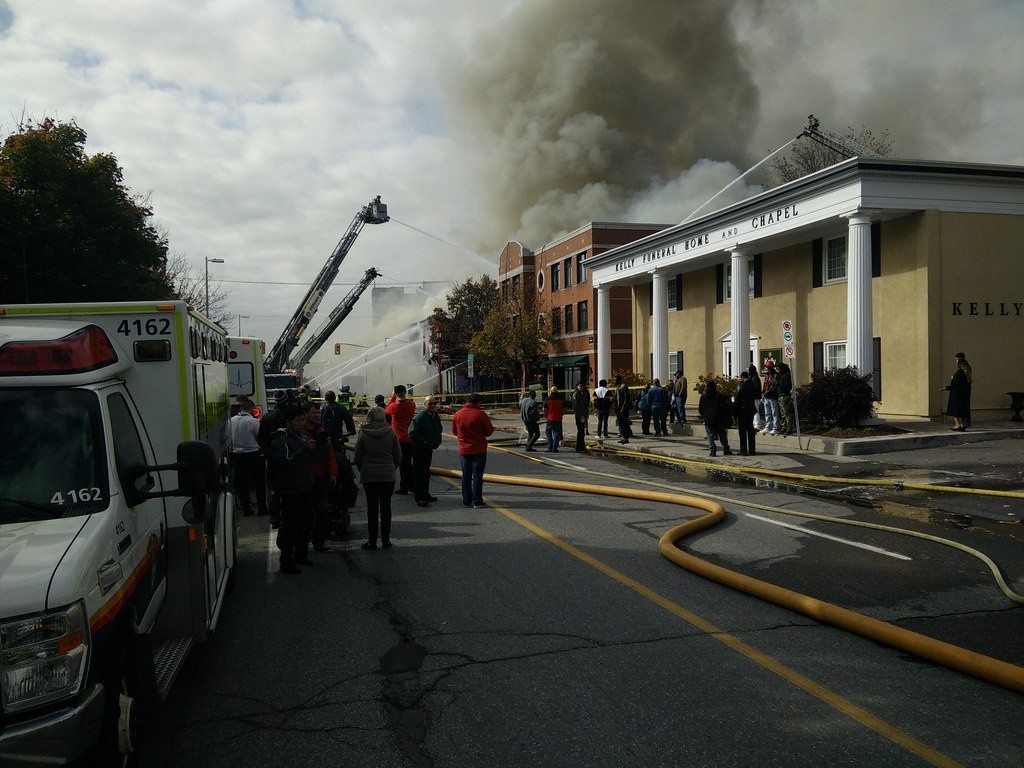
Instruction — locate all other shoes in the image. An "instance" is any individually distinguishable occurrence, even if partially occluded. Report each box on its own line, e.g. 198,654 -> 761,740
950,426 -> 966,432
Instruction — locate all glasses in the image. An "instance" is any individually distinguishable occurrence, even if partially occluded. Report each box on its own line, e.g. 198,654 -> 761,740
424,401 -> 438,406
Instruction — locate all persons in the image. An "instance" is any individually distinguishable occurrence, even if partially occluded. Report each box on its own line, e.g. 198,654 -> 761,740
698,361 -> 792,456
265,405 -> 315,574
293,402 -> 338,554
384,385 -> 417,495
950,353 -> 971,428
229,383 -> 356,530
591,379 -> 614,438
408,396 -> 443,506
637,370 -> 687,436
612,378 -> 633,437
354,407 -> 402,550
451,394 -> 494,509
572,383 -> 588,453
542,386 -> 565,452
571,382 -> 590,435
521,390 -> 541,451
939,359 -> 972,432
614,374 -> 629,444
374,394 -> 391,424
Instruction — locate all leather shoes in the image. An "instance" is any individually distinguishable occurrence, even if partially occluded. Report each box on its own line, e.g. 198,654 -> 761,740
576,449 -> 589,453
618,430 -> 633,444
777,427 -> 793,435
526,448 -> 537,452
280,557 -> 315,574
724,449 -> 732,455
544,447 -> 559,453
654,432 -> 668,436
737,449 -> 756,456
709,451 -> 716,457
417,496 -> 438,507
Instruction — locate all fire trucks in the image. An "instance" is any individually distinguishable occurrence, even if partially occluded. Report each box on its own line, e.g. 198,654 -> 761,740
265,196 -> 390,408
217,337 -> 269,425
0,299 -> 236,766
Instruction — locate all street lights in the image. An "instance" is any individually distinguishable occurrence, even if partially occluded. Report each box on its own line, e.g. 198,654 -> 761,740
238,315 -> 249,337
204,256 -> 225,320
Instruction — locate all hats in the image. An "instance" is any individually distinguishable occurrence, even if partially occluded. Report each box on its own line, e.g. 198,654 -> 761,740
375,394 -> 385,403
274,390 -> 289,405
766,361 -> 774,368
674,370 -> 682,375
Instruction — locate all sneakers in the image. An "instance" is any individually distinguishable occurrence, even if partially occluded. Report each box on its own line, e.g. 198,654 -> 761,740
361,541 -> 391,550
759,428 -> 779,434
464,502 -> 487,508
595,434 -> 611,439
242,509 -> 268,516
314,545 -> 332,553
